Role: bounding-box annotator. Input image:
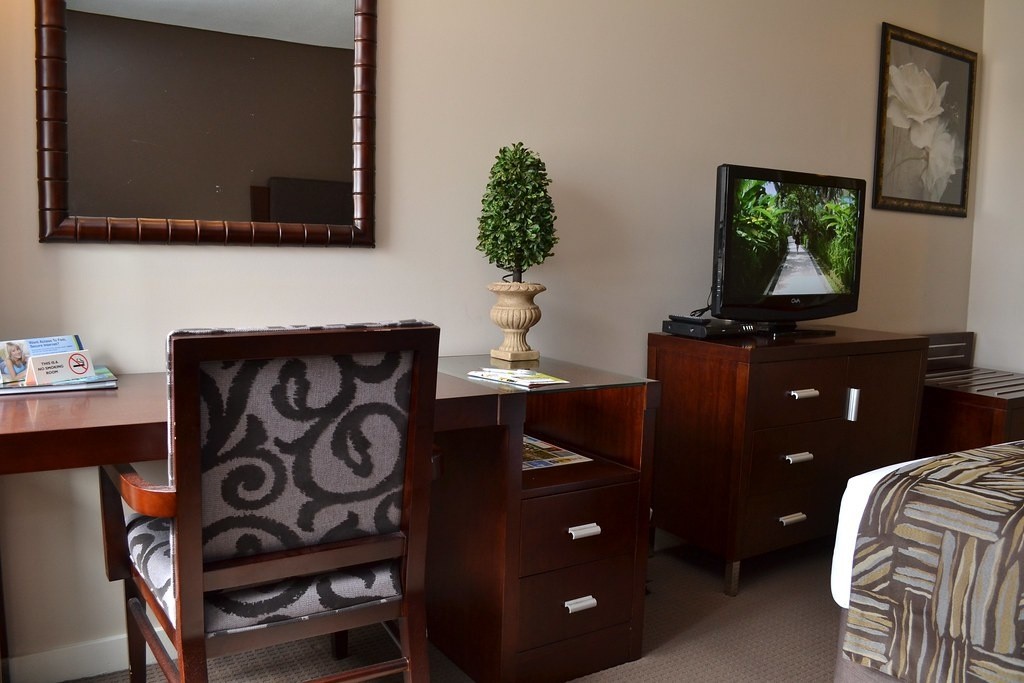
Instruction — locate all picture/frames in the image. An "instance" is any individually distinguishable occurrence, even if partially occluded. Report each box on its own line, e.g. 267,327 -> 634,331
871,21 -> 977,226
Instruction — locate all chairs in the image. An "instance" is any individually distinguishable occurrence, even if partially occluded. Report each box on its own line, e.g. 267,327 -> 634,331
97,320 -> 441,682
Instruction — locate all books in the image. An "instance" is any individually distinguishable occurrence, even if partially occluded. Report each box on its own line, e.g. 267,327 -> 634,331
0,364 -> 118,396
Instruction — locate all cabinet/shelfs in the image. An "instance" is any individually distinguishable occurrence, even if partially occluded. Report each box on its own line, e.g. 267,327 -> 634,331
915,331 -> 1024,459
646,327 -> 929,598
429,350 -> 661,683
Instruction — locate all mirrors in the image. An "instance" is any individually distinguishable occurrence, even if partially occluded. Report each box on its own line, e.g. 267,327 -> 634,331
34,0 -> 379,251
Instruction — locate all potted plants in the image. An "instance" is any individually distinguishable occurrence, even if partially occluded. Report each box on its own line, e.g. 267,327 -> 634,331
475,143 -> 560,361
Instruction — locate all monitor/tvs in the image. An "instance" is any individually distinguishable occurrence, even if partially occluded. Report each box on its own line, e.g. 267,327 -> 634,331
710,165 -> 866,338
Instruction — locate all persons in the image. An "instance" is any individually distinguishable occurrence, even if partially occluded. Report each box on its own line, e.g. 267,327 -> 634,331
0,341 -> 31,380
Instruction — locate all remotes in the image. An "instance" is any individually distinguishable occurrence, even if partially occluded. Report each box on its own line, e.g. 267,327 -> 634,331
669,314 -> 710,324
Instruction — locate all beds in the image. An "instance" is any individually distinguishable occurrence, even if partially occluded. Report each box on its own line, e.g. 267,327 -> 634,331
831,431 -> 1024,683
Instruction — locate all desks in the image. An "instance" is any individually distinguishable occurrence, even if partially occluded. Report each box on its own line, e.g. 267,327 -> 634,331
1,372 -> 501,475
